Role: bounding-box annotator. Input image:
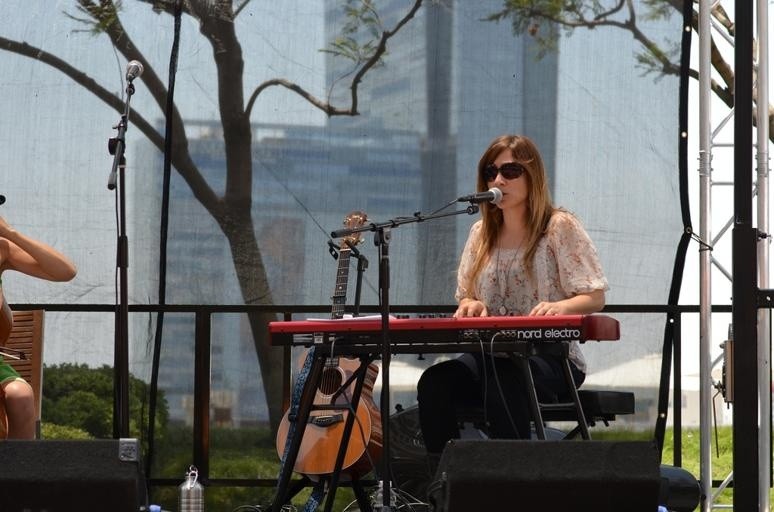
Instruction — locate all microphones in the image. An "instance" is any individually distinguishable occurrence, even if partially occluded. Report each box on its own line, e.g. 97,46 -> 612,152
457,187 -> 503,204
126,59 -> 145,83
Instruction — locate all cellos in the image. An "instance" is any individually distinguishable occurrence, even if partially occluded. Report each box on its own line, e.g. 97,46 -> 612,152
0,195 -> 11,438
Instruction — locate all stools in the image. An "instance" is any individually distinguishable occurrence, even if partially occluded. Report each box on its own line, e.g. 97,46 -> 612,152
540,389 -> 635,442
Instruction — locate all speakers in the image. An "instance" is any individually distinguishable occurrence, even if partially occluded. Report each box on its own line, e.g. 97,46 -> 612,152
429,439 -> 663,512
0,437 -> 149,512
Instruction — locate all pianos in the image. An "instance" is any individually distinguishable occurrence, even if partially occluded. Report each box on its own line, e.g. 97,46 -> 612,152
268,314 -> 621,347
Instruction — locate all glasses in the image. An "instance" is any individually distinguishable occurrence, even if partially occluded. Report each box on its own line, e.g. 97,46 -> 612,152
483,162 -> 523,180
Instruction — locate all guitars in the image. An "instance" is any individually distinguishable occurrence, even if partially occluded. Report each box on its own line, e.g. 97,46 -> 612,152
276,210 -> 385,483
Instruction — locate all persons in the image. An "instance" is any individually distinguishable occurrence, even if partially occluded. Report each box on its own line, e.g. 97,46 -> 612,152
416,135 -> 611,481
0,194 -> 79,441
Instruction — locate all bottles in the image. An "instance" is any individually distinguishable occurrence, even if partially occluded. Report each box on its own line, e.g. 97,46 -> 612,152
372,480 -> 397,512
178,465 -> 204,512
